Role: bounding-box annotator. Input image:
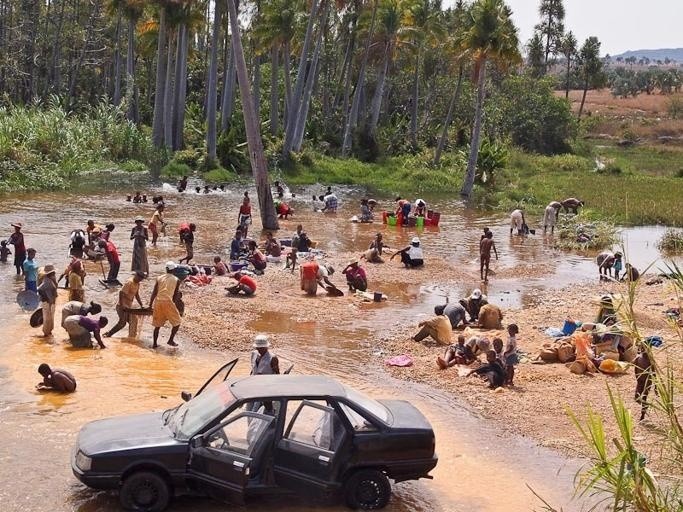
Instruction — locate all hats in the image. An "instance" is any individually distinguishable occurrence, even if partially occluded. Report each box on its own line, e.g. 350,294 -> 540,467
412,237 -> 420,243
135,215 -> 145,223
472,288 -> 482,299
136,270 -> 145,278
347,258 -> 359,265
252,335 -> 269,349
11,223 -> 22,228
37,264 -> 57,275
165,261 -> 179,270
325,265 -> 335,276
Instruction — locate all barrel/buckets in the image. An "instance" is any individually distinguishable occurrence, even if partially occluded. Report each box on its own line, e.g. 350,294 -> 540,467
416,217 -> 424,226
374,292 -> 382,302
388,215 -> 397,226
424,218 -> 432,226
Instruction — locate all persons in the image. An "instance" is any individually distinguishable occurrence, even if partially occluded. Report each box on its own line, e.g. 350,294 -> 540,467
559,197 -> 584,215
34,362 -> 75,394
390,237 -> 424,269
597,253 -> 613,281
410,288 -> 517,389
246,334 -> 276,423
478,231 -> 498,280
509,208 -> 528,233
619,262 -> 638,282
0,220 -> 389,352
613,252 -> 622,281
592,295 -> 617,340
115,175 -> 441,225
631,338 -> 655,419
542,200 -> 562,233
479,227 -> 490,259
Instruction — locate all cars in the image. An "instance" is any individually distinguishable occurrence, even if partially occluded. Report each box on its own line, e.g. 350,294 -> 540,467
70,357 -> 439,510
